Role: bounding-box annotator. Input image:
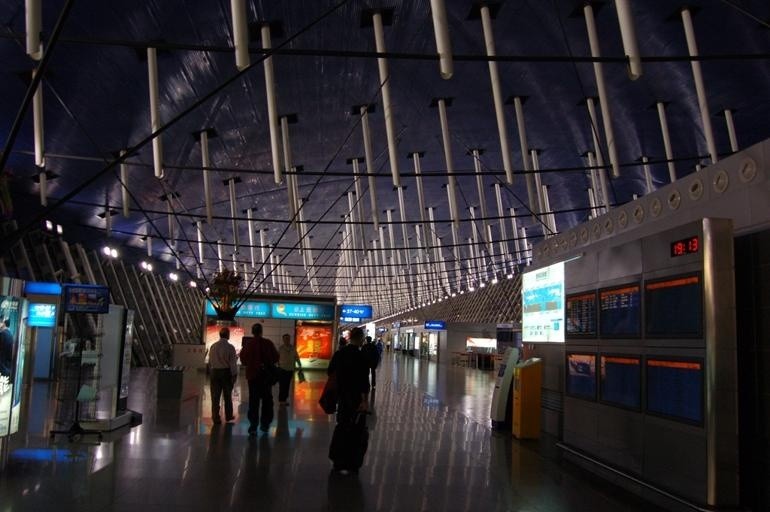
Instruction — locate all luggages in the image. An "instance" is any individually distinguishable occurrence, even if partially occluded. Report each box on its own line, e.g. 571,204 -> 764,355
328,411 -> 372,472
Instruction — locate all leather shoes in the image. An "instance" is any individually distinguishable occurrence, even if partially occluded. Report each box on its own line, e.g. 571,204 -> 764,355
226,416 -> 235,421
212,415 -> 221,424
248,423 -> 270,435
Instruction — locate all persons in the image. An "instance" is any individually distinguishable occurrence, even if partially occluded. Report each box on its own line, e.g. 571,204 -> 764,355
328,328 -> 370,423
240,324 -> 280,436
0,315 -> 14,383
209,328 -> 238,424
361,336 -> 382,390
276,334 -> 302,406
339,338 -> 346,350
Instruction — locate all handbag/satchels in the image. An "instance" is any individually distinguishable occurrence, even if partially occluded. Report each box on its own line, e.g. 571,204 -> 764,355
260,367 -> 279,390
319,372 -> 336,414
